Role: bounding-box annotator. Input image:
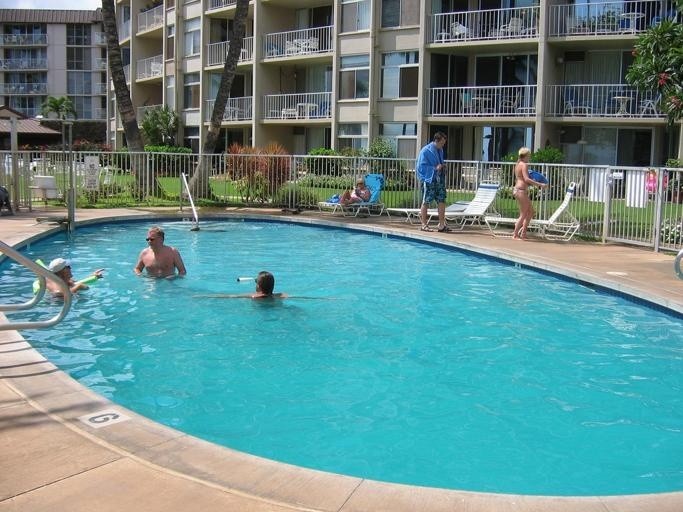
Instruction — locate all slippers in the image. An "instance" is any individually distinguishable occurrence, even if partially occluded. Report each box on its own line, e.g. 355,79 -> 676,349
421,225 -> 433,231
438,226 -> 452,232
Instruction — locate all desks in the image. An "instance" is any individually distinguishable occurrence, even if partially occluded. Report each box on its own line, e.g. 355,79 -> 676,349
612,95 -> 635,114
471,96 -> 494,114
621,11 -> 645,35
437,33 -> 449,42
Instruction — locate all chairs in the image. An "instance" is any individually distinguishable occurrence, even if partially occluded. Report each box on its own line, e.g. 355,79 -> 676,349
448,20 -> 473,41
565,14 -> 589,36
30,174 -> 63,206
316,174 -> 386,219
458,92 -> 476,114
484,182 -> 580,242
500,17 -> 521,36
496,90 -> 521,114
387,179 -> 501,237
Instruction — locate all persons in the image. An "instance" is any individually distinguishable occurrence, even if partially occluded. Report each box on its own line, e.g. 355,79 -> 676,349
338,178 -> 370,204
251,270 -> 288,299
512,147 -> 548,241
41,258 -> 104,296
135,227 -> 186,279
416,131 -> 453,233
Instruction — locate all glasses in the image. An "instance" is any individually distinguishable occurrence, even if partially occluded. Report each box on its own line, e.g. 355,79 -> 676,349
66,265 -> 71,269
357,183 -> 363,185
255,278 -> 257,284
146,238 -> 155,241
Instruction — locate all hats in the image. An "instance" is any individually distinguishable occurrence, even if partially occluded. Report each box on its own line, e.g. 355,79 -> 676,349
49,258 -> 67,273
